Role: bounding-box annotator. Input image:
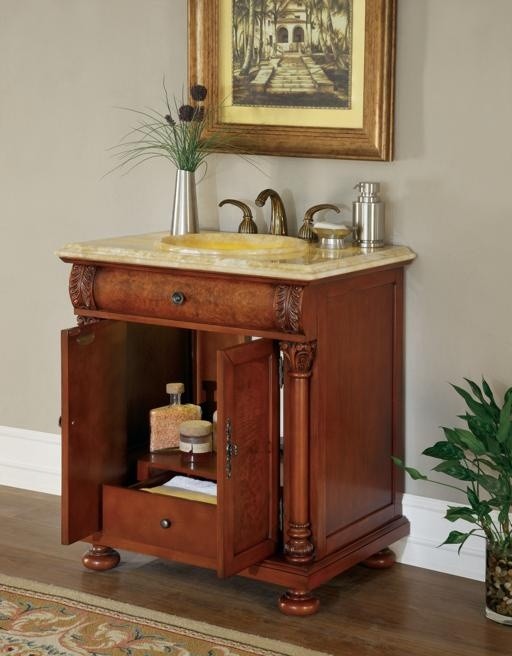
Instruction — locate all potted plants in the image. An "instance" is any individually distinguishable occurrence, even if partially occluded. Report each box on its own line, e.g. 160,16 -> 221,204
102,74 -> 271,235
392,375 -> 512,626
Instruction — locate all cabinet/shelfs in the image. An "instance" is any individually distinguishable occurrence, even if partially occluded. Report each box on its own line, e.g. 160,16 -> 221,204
60,264 -> 412,617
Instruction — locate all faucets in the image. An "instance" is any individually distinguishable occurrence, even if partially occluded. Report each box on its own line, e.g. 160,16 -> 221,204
255,189 -> 288,235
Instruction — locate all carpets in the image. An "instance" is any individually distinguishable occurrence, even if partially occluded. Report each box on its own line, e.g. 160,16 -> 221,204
0,574 -> 333,656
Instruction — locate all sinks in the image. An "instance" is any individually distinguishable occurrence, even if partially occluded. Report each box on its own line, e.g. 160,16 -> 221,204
154,232 -> 308,255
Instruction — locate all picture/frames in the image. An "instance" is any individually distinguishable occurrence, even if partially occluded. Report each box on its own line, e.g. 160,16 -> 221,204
187,0 -> 398,162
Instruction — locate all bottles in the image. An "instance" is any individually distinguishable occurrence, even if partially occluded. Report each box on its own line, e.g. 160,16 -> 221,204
352,182 -> 385,249
149,383 -> 216,462
